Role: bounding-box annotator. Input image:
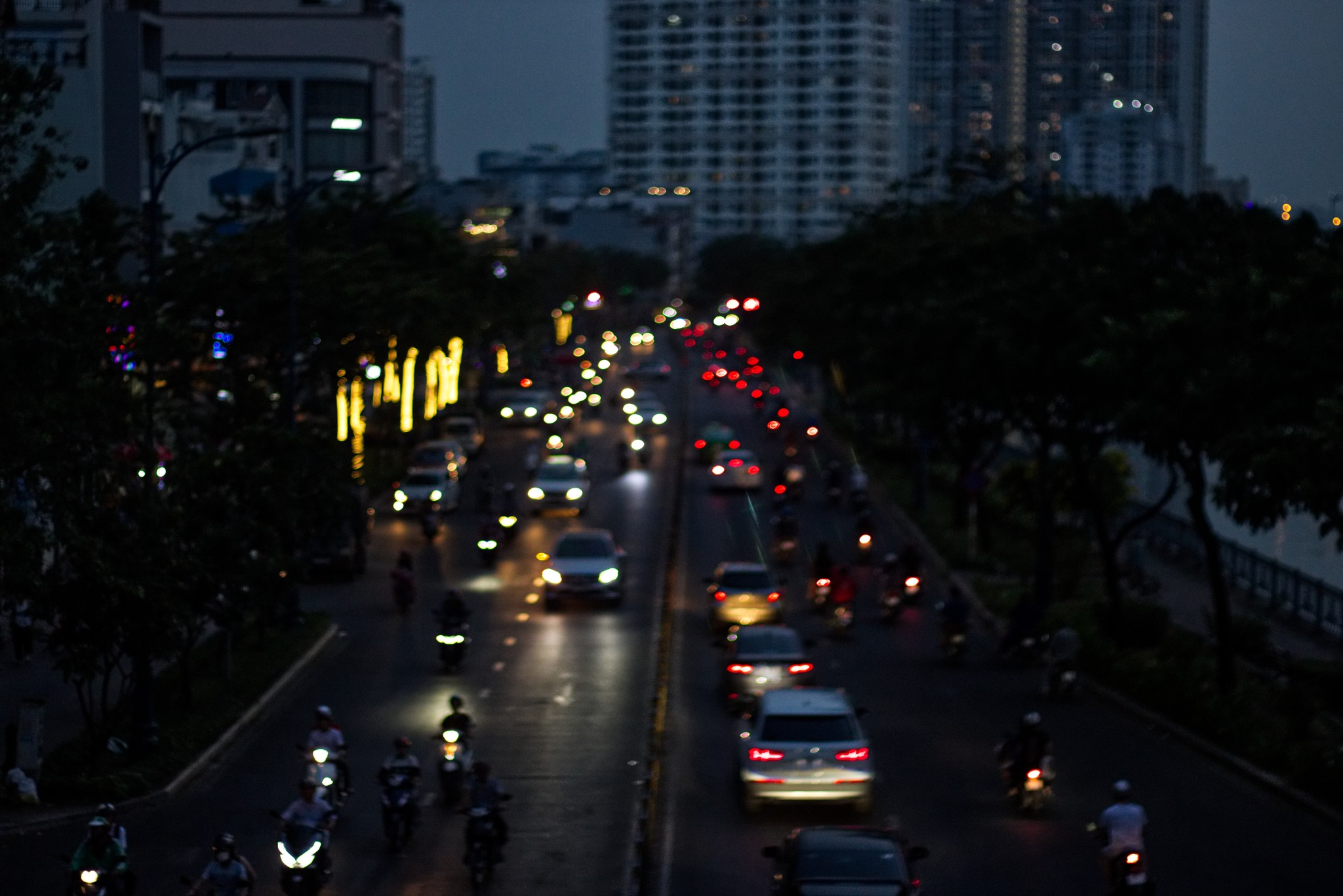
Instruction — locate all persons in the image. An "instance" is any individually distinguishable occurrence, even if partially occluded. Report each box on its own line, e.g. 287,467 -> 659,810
438,588 -> 469,657
279,779 -> 337,875
470,758 -> 509,855
70,804 -> 128,881
396,552 -> 413,573
441,696 -> 474,771
901,540 -> 920,576
383,734 -> 419,802
1001,711 -> 1050,787
812,540 -> 837,580
1045,614 -> 1077,690
776,506 -> 799,539
938,585 -> 970,636
995,591 -> 1046,655
307,705 -> 354,796
186,833 -> 257,896
829,567 -> 856,603
855,509 -> 877,534
1095,780 -> 1148,884
882,553 -> 903,587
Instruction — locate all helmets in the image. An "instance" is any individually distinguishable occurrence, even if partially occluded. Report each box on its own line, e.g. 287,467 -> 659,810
315,704 -> 332,717
472,759 -> 491,775
394,737 -> 411,747
299,777 -> 315,789
94,803 -> 116,816
214,833 -> 235,847
87,818 -> 112,833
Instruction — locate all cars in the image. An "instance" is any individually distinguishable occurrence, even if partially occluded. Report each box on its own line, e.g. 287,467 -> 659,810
760,824 -> 930,896
390,349 -> 1080,705
730,689 -> 875,819
712,625 -> 816,712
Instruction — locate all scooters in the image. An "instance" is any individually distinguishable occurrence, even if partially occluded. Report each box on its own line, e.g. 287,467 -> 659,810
267,805 -> 340,896
998,711 -> 1055,822
446,789 -> 511,891
1084,817 -> 1159,896
60,852 -> 136,896
181,870 -> 259,896
372,766 -> 423,848
289,738 -> 356,813
428,722 -> 477,810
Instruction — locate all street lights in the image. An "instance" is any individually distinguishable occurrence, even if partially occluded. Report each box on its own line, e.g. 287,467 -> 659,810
137,114 -> 366,762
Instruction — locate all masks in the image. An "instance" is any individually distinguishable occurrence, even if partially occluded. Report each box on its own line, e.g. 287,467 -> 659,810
216,852 -> 229,862
